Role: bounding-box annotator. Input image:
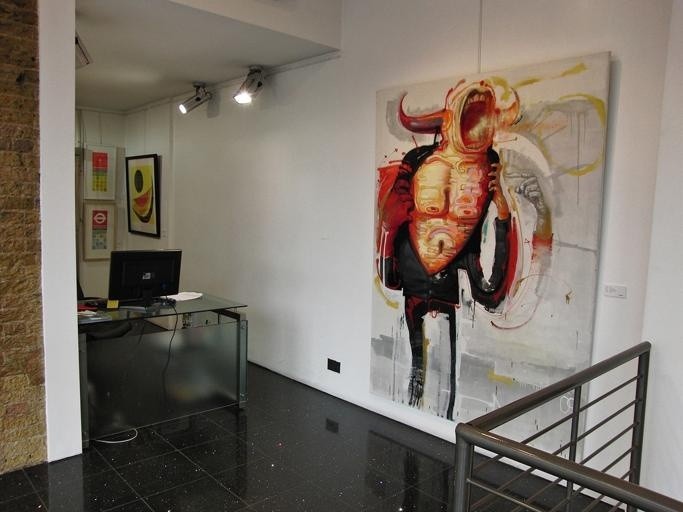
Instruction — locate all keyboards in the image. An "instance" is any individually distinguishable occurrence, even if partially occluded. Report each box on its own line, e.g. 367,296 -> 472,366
85,299 -> 176,311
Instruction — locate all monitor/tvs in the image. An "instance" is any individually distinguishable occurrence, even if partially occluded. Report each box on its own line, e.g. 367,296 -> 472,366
108,250 -> 182,308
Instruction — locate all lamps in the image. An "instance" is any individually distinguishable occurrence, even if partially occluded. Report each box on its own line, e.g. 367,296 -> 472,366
178,80 -> 213,115
232,63 -> 268,107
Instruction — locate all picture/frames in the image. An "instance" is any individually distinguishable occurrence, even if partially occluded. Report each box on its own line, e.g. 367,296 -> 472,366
81,201 -> 118,263
124,153 -> 161,239
82,143 -> 118,202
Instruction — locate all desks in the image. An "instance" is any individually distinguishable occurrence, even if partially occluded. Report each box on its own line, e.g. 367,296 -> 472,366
76,290 -> 248,449
